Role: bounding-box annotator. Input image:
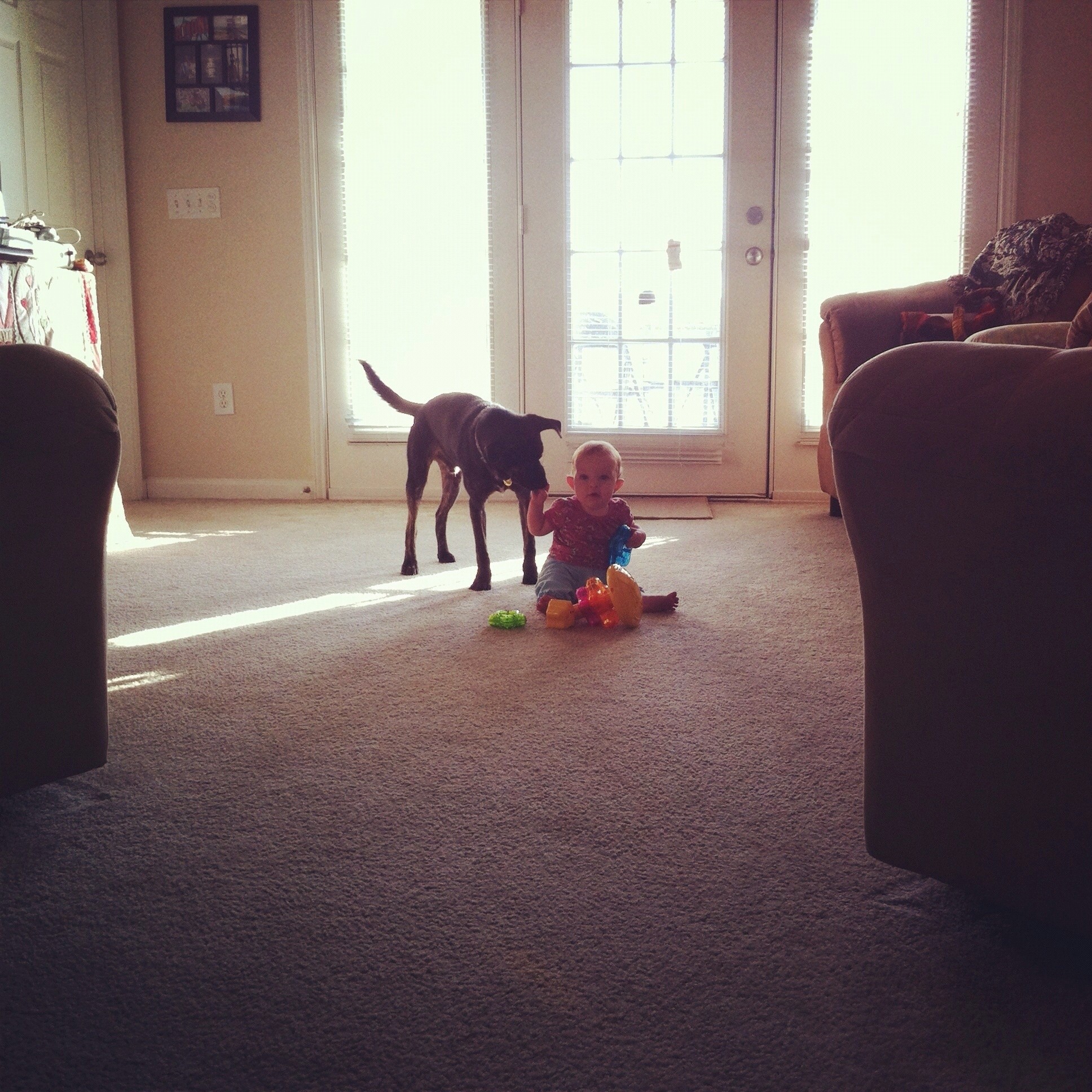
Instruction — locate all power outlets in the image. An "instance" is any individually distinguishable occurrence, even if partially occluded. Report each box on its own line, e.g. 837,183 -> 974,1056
214,383 -> 235,415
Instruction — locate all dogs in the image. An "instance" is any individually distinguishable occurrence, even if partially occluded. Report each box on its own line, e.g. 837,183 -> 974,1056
358,360 -> 562,591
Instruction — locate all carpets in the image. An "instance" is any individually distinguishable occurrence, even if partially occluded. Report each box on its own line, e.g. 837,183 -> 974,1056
626,496 -> 714,520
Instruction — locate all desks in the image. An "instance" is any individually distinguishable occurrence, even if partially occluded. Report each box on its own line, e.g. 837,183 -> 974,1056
0,260 -> 104,378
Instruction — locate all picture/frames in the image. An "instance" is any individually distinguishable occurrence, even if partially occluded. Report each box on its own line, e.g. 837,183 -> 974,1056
164,6 -> 261,123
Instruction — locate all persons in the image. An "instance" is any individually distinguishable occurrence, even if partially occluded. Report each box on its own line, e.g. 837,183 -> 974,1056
526,440 -> 679,615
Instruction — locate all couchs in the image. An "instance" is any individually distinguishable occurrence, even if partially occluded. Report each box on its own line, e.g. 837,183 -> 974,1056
0,343 -> 122,788
817,214 -> 1092,907
